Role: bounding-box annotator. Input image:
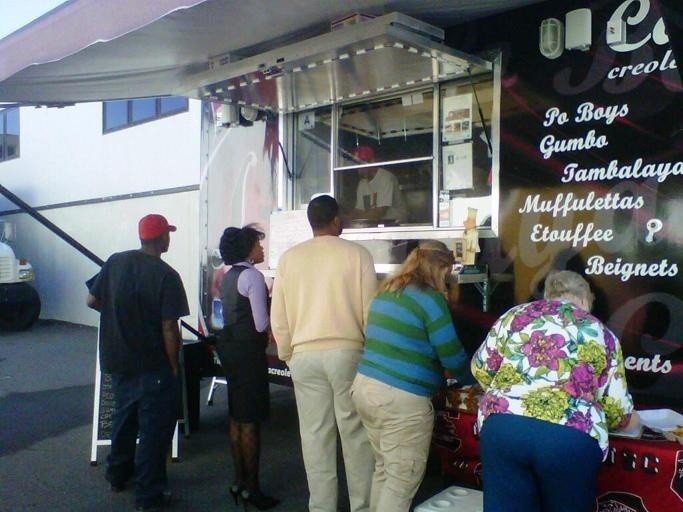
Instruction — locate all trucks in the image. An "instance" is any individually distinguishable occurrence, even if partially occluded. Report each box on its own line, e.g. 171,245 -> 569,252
0,243 -> 41,330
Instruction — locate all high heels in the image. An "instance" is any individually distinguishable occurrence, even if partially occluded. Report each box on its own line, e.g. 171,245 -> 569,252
240,488 -> 279,511
228,484 -> 244,506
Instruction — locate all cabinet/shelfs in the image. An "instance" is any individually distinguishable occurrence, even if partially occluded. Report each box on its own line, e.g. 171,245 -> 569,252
169,337 -> 205,433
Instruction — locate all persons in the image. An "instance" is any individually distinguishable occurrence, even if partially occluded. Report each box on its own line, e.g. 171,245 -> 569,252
340,142 -> 411,227
269,193 -> 380,512
468,268 -> 644,511
216,222 -> 282,510
348,237 -> 480,512
84,213 -> 190,510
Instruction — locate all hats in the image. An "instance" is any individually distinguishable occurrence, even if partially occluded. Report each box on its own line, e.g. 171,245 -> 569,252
138,214 -> 176,241
353,146 -> 375,164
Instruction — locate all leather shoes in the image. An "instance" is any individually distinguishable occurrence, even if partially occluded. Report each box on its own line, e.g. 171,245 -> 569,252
135,489 -> 172,511
105,479 -> 131,491
